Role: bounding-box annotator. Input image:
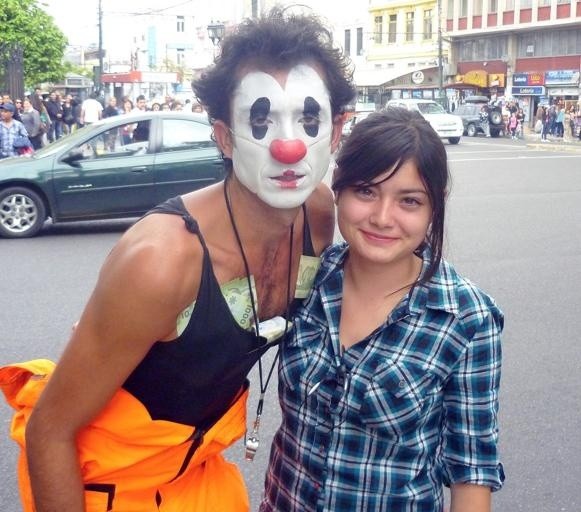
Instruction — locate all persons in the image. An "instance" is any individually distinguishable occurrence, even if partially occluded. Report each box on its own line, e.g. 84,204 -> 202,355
478,101 -> 581,142
257,106 -> 505,511
0,87 -> 209,158
1,2 -> 356,512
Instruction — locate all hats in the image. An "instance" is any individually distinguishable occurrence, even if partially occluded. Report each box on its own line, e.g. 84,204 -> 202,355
0,104 -> 16,113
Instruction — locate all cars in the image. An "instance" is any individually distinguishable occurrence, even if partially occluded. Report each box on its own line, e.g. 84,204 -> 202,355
336,111 -> 373,151
0,107 -> 224,242
144,94 -> 187,111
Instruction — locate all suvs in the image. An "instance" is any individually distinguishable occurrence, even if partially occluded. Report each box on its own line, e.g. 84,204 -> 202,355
451,95 -> 506,138
382,98 -> 465,147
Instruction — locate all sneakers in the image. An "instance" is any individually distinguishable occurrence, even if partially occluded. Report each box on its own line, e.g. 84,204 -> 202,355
512,135 -> 518,141
542,135 -> 574,144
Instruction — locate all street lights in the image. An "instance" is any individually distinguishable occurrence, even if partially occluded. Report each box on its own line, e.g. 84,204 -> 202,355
206,17 -> 227,65
